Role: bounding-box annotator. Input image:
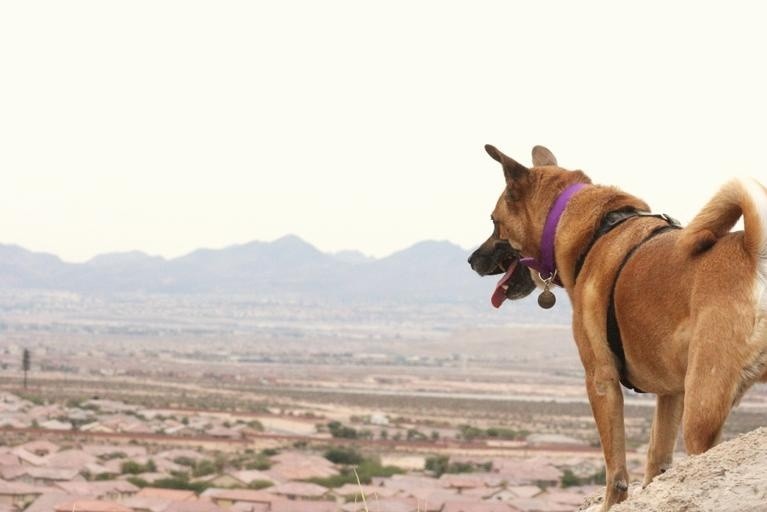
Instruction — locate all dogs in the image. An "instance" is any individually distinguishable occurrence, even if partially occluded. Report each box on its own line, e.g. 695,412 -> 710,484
467,143 -> 767,512
